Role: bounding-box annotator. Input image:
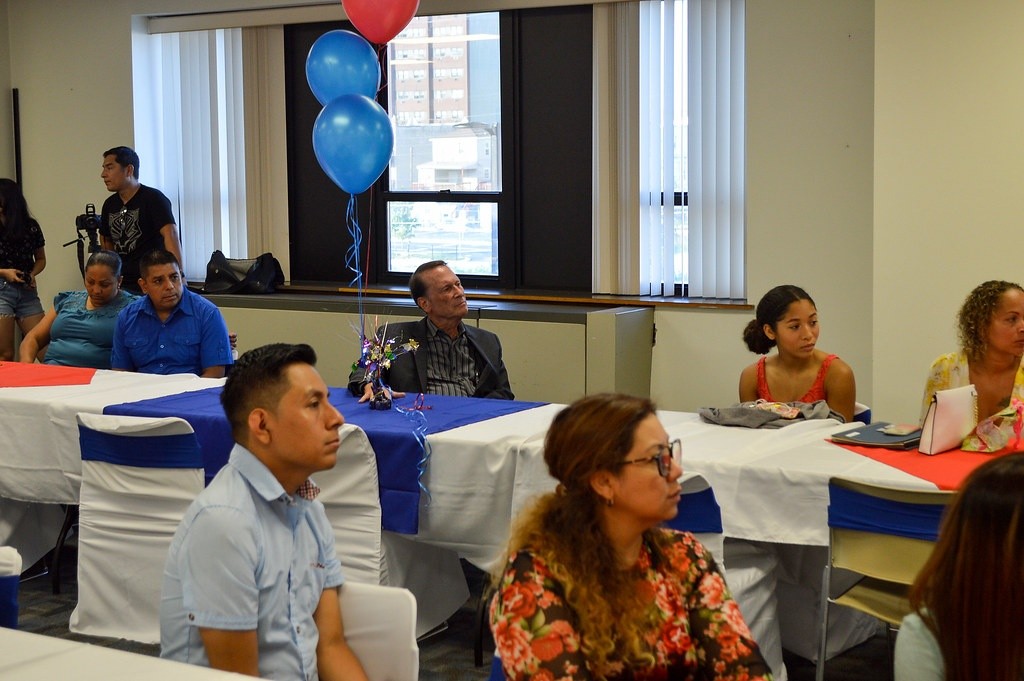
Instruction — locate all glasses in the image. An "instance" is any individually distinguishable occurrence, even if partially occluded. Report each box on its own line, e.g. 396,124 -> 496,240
599,438 -> 682,477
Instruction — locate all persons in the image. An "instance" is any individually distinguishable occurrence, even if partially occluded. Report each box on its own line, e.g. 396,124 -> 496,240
110,249 -> 234,378
347,261 -> 515,403
484,393 -> 772,681
0,178 -> 49,361
918,277 -> 1024,451
159,343 -> 370,681
894,452 -> 1024,681
19,251 -> 142,369
740,285 -> 855,422
99,146 -> 187,286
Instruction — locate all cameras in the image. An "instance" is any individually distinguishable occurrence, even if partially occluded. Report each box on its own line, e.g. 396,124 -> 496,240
76,204 -> 102,231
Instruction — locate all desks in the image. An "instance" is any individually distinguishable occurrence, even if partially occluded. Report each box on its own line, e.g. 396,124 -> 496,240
0,360 -> 1024,681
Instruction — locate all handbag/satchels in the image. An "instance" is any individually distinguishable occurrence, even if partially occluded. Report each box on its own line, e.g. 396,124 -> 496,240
919,385 -> 980,455
188,251 -> 286,295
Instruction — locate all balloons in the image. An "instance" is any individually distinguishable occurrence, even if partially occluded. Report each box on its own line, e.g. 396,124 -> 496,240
306,30 -> 381,105
313,93 -> 394,201
341,0 -> 420,46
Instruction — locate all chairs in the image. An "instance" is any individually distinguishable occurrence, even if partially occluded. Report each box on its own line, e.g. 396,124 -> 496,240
815,476 -> 956,681
310,426 -> 471,648
0,546 -> 23,630
67,414 -> 205,644
339,581 -> 420,681
664,471 -> 787,681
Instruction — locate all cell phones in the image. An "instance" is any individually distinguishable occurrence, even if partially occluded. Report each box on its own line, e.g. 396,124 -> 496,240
885,423 -> 920,436
